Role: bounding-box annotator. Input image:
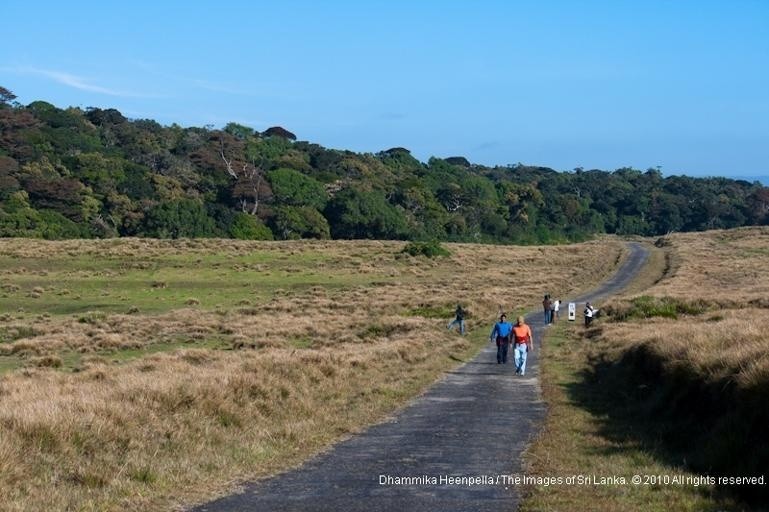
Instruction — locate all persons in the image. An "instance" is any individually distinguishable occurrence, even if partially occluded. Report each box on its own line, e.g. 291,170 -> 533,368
490,315 -> 513,365
446,305 -> 468,338
509,316 -> 534,376
543,294 -> 562,326
584,302 -> 595,326
